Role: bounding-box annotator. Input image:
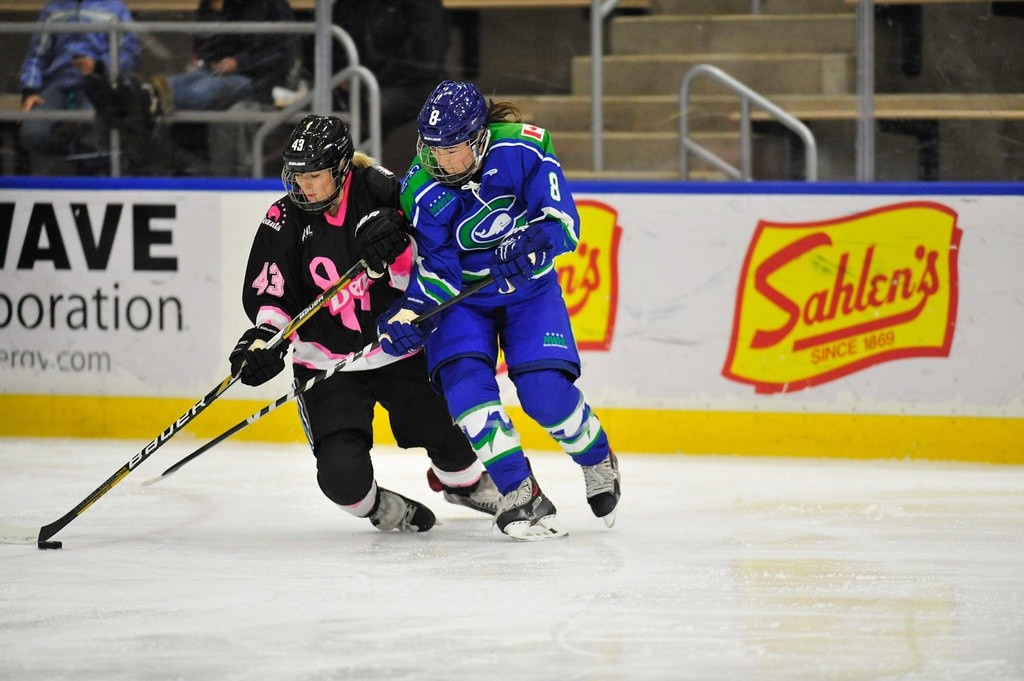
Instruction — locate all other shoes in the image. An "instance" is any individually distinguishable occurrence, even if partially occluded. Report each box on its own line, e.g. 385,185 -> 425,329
86,73 -> 156,140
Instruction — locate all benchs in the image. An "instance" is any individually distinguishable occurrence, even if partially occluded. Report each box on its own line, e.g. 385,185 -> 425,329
739,111 -> 1024,180
0,0 -> 313,181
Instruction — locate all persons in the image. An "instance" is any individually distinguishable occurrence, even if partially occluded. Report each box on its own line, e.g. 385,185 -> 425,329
19,0 -> 154,175
86,0 -> 301,138
229,114 -> 505,533
376,79 -> 621,542
263,0 -> 450,146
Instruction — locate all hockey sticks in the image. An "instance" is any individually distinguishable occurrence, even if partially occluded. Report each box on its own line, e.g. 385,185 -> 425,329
35,259 -> 374,549
141,273 -> 502,484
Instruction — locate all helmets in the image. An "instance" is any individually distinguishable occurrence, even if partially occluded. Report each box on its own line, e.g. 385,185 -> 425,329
416,79 -> 491,189
281,114 -> 355,215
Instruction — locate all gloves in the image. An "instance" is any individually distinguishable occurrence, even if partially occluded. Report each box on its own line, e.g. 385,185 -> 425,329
490,225 -> 553,291
355,208 -> 411,279
228,323 -> 291,387
376,294 -> 447,357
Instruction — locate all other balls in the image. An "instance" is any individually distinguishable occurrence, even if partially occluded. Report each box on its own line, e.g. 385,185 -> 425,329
37,540 -> 63,551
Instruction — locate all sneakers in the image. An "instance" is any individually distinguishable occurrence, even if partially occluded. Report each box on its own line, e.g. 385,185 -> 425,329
369,486 -> 443,533
427,466 -> 504,515
491,456 -> 569,543
582,448 -> 620,528
